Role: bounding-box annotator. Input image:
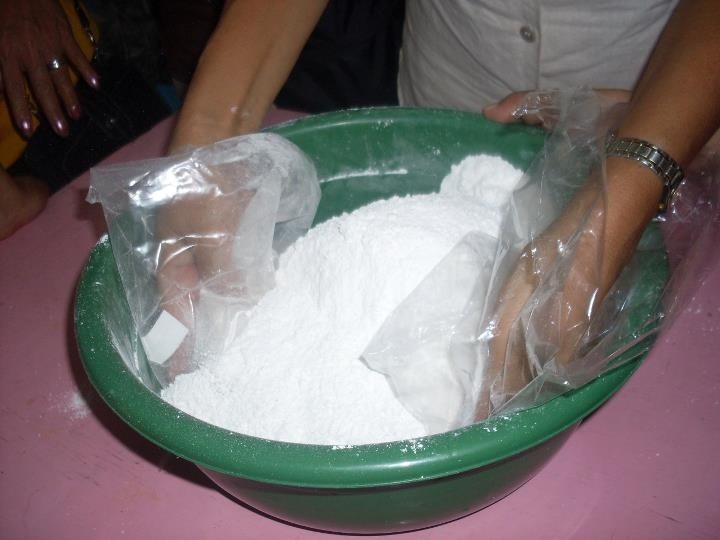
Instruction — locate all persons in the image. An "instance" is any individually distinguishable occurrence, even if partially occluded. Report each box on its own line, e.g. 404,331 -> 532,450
483,88 -> 720,178
0,0 -> 101,242
155,0 -> 720,426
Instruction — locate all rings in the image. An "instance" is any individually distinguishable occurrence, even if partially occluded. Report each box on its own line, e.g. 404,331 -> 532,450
47,58 -> 66,71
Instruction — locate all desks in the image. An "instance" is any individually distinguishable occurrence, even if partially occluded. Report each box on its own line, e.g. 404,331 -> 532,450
1,107 -> 720,540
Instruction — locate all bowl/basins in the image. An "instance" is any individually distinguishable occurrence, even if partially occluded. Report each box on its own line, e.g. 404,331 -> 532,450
71,104 -> 675,536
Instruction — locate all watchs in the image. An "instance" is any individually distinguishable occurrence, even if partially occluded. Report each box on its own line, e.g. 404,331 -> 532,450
603,137 -> 688,217
